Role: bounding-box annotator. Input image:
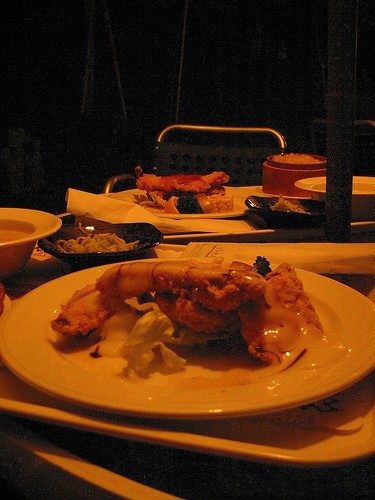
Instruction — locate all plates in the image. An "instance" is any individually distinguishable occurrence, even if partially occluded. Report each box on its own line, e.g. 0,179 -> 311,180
151,188 -> 252,220
0,257 -> 375,421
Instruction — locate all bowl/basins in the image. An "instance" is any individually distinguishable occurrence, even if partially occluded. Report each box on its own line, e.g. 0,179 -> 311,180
295,175 -> 375,220
37,216 -> 164,269
244,195 -> 326,228
0,207 -> 63,277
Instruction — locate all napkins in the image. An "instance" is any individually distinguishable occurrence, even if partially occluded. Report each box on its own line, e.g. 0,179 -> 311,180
143,241 -> 375,276
64,188 -> 256,234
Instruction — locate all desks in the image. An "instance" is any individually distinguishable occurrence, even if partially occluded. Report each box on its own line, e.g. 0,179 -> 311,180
0,209 -> 375,500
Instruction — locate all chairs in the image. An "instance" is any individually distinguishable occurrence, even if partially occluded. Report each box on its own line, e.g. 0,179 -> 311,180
310,118 -> 375,176
103,123 -> 287,194
